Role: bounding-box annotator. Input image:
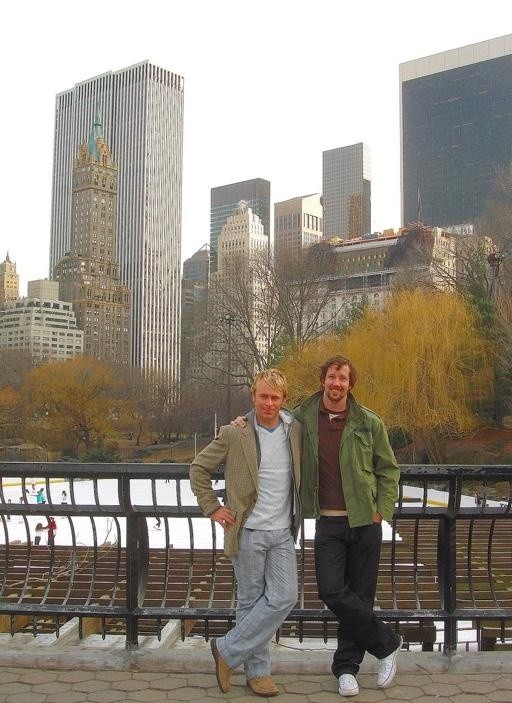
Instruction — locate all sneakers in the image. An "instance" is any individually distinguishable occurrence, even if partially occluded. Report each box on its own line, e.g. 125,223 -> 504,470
338,674 -> 359,697
376,635 -> 404,688
247,677 -> 279,697
210,639 -> 234,693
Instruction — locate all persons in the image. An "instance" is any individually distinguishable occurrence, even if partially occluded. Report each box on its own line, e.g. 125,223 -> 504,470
189,370 -> 304,698
0,483 -> 68,546
229,357 -> 404,696
154,516 -> 161,530
